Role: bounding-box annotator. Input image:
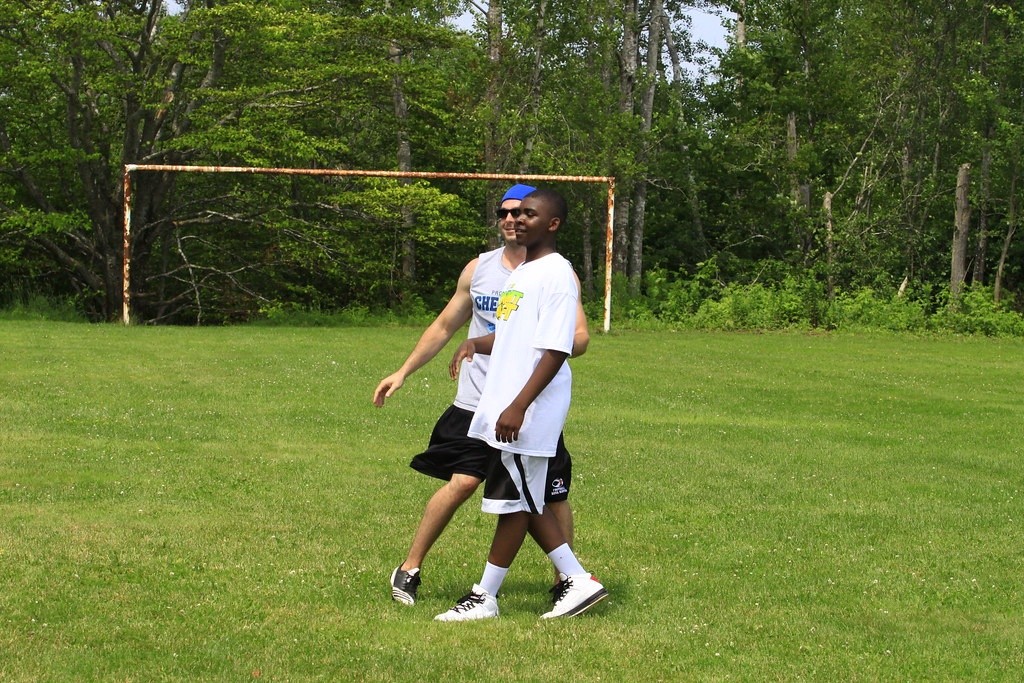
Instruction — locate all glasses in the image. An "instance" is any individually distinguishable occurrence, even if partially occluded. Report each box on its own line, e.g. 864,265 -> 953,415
496,207 -> 521,219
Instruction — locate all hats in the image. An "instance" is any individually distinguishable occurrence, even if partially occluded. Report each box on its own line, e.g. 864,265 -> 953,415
501,184 -> 537,203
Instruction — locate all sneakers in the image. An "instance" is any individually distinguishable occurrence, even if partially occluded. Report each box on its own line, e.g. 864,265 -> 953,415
391,560 -> 423,605
432,583 -> 500,621
538,573 -> 610,621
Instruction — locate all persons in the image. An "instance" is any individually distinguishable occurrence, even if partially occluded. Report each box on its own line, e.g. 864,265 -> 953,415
431,189 -> 610,624
372,183 -> 589,607
